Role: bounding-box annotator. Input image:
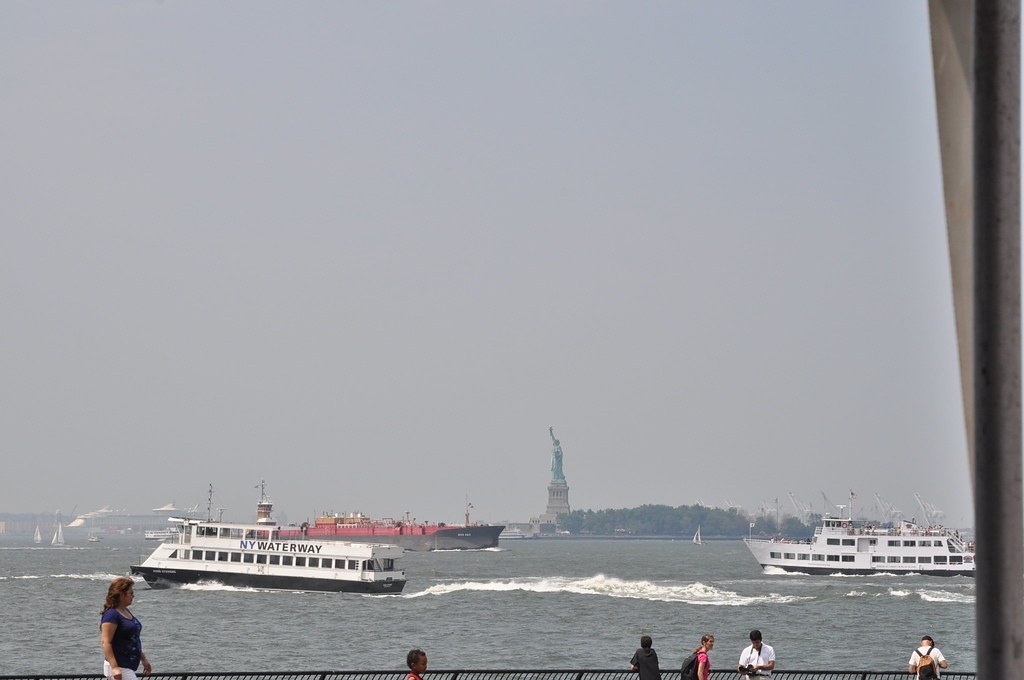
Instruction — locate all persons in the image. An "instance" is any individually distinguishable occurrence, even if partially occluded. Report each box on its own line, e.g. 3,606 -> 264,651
100,576 -> 152,680
406,649 -> 428,680
771,537 -> 811,544
908,636 -> 947,680
848,522 -> 976,553
549,427 -> 567,483
692,634 -> 714,680
735,630 -> 775,680
629,636 -> 662,680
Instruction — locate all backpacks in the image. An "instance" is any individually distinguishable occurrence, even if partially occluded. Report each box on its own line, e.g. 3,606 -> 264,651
914,646 -> 941,680
681,647 -> 708,680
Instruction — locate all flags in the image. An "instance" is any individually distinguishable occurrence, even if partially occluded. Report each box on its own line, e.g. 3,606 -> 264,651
750,522 -> 755,528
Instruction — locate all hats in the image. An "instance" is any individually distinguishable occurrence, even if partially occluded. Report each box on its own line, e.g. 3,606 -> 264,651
921,636 -> 935,647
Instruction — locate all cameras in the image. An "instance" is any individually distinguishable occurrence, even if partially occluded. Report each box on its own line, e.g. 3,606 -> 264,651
739,665 -> 756,676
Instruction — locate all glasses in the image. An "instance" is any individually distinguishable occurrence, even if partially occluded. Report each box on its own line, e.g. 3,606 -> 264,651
708,640 -> 714,643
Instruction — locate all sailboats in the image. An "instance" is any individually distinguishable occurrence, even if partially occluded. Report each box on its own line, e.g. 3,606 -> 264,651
87,515 -> 104,542
51,522 -> 65,547
34,525 -> 41,544
692,524 -> 702,546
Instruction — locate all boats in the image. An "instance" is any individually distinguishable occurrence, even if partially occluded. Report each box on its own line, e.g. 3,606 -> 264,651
742,487 -> 978,579
260,493 -> 506,551
128,479 -> 411,595
144,517 -> 205,540
